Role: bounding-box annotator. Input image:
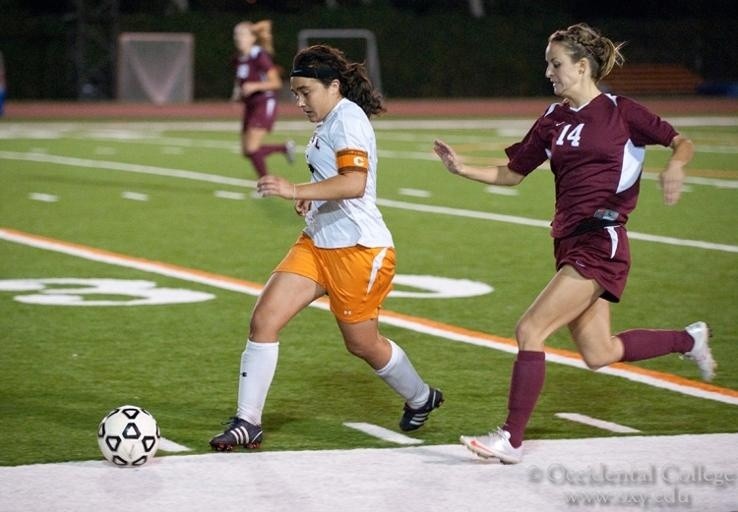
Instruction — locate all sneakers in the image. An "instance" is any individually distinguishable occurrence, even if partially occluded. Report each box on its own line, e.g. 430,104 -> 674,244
400,385 -> 444,431
460,427 -> 523,463
285,141 -> 295,163
683,320 -> 716,380
210,416 -> 263,450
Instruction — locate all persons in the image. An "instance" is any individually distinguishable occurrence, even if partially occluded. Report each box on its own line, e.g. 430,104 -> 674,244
229,18 -> 296,199
431,25 -> 719,465
209,44 -> 446,451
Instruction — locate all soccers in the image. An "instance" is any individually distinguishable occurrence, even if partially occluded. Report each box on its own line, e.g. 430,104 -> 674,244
97,406 -> 161,468
